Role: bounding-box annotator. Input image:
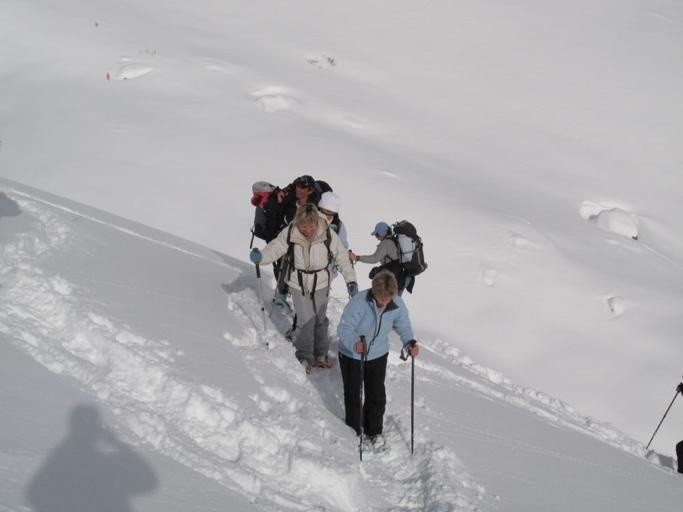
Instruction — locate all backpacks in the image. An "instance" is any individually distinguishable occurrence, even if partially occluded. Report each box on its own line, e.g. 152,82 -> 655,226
250,180 -> 282,241
385,219 -> 427,277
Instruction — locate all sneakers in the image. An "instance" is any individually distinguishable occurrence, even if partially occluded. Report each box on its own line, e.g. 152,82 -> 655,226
298,356 -> 333,374
354,432 -> 387,454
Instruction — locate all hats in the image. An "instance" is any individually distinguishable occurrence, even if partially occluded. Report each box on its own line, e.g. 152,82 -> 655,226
294,174 -> 315,188
370,221 -> 391,238
317,191 -> 342,214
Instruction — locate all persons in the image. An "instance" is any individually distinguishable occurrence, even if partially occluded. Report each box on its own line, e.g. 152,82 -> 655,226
266,174 -> 315,307
674,382 -> 683,474
279,190 -> 350,341
249,200 -> 359,373
346,222 -> 411,295
332,268 -> 419,451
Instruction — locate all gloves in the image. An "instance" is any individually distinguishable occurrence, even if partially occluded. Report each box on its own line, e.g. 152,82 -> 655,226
249,247 -> 263,264
347,282 -> 359,298
